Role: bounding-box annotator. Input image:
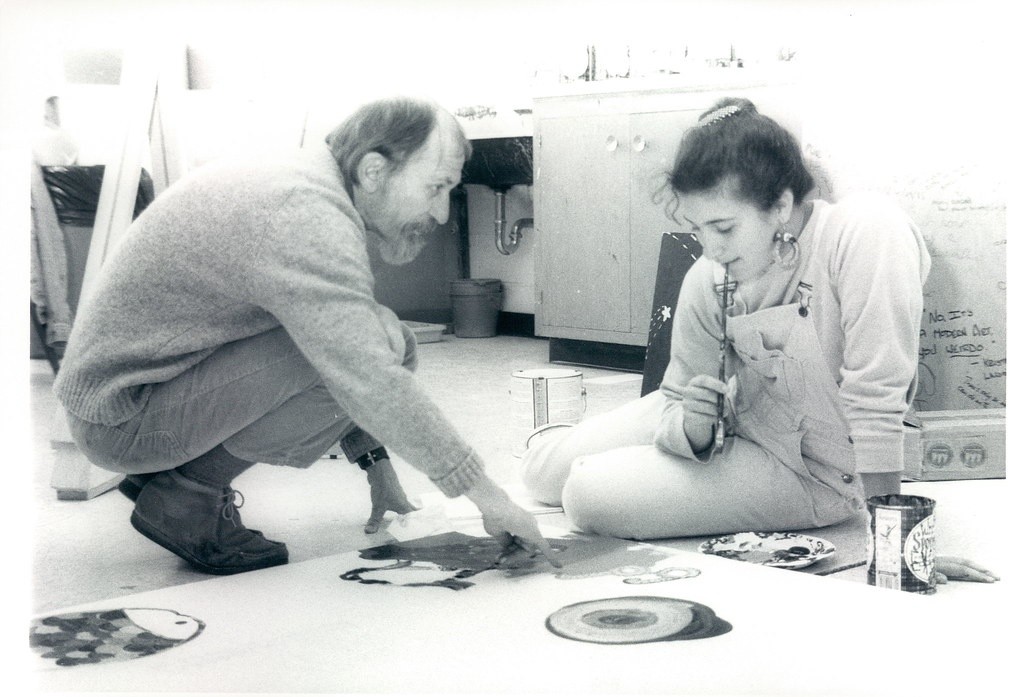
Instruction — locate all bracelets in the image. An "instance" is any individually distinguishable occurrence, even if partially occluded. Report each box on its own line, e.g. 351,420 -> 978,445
357,446 -> 389,470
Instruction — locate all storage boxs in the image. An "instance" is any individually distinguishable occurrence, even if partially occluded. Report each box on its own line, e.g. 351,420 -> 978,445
902,408 -> 1007,481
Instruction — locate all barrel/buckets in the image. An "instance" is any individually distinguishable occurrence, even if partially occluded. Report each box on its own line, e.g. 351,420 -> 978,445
508,368 -> 586,459
450,278 -> 504,337
867,494 -> 938,595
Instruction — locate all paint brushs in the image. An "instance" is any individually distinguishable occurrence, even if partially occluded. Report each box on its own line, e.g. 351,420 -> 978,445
715,263 -> 730,454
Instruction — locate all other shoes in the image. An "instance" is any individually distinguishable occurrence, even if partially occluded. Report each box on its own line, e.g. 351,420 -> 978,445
119,467 -> 289,575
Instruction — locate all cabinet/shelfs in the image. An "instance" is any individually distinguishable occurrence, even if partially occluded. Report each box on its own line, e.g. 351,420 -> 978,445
532,69 -> 779,381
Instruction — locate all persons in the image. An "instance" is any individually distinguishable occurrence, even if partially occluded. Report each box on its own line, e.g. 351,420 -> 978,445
52,96 -> 564,576
523,98 -> 1000,585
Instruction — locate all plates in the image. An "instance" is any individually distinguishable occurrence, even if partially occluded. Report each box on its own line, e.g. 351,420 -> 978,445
698,531 -> 836,570
